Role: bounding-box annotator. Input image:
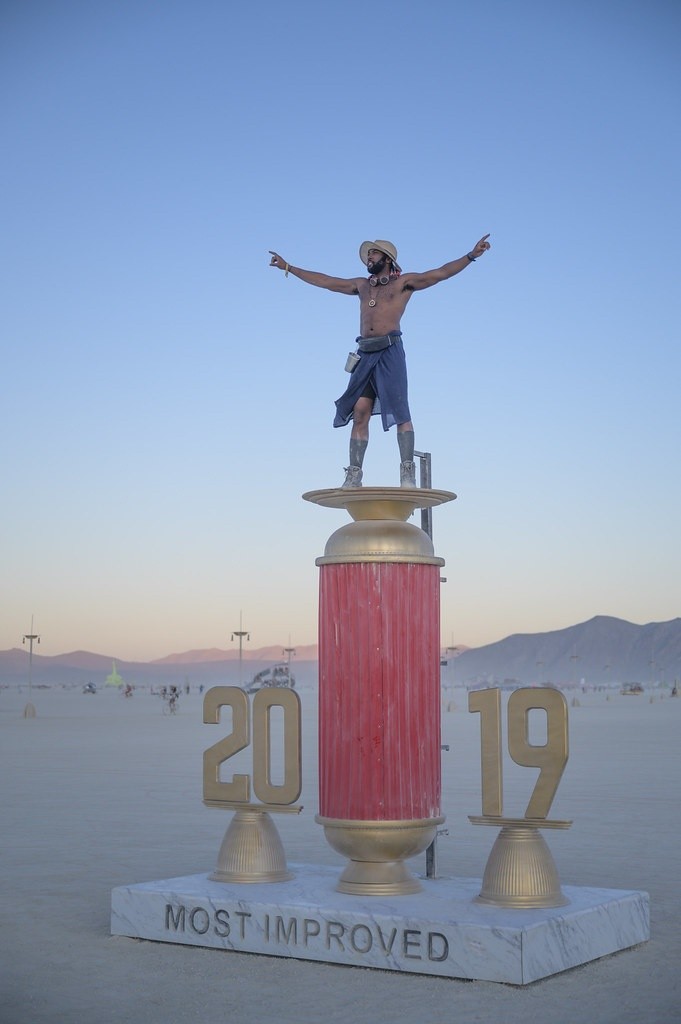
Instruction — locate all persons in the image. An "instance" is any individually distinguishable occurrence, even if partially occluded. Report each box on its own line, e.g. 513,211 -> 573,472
169,687 -> 179,709
269,233 -> 491,488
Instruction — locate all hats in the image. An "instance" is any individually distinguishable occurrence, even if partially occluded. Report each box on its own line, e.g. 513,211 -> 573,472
359,239 -> 402,273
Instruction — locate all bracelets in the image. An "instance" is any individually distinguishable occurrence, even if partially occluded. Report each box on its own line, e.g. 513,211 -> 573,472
467,252 -> 476,262
285,263 -> 293,278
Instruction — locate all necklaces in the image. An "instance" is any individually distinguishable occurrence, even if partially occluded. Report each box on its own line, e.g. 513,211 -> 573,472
369,285 -> 381,307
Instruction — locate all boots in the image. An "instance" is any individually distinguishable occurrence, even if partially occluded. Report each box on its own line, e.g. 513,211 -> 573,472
341,464 -> 363,486
400,460 -> 416,487
